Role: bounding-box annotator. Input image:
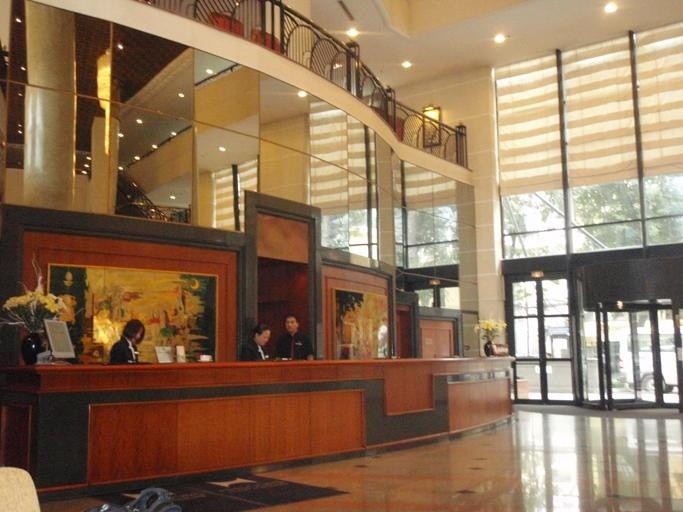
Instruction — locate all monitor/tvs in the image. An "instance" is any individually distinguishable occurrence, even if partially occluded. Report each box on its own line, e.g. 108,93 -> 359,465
44,319 -> 76,359
293,343 -> 304,360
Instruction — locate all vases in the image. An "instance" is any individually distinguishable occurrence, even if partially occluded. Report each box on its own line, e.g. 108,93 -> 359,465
0,291 -> 69,334
20,334 -> 41,366
484,341 -> 497,356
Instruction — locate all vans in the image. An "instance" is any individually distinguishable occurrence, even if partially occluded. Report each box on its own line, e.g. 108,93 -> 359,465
617,321 -> 682,394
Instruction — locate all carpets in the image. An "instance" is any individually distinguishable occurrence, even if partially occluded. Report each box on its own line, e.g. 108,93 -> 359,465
89,472 -> 352,511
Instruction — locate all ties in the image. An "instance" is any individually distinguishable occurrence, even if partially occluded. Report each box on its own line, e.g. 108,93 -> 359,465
290,337 -> 294,359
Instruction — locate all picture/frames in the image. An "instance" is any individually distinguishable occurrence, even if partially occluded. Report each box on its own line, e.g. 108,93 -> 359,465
331,287 -> 389,359
423,107 -> 441,148
47,262 -> 219,364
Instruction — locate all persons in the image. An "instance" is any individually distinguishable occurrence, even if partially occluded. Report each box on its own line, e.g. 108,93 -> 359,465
240,323 -> 273,362
274,314 -> 315,361
106,318 -> 145,364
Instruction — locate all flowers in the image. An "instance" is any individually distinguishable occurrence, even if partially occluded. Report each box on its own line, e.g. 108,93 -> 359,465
474,319 -> 508,341
